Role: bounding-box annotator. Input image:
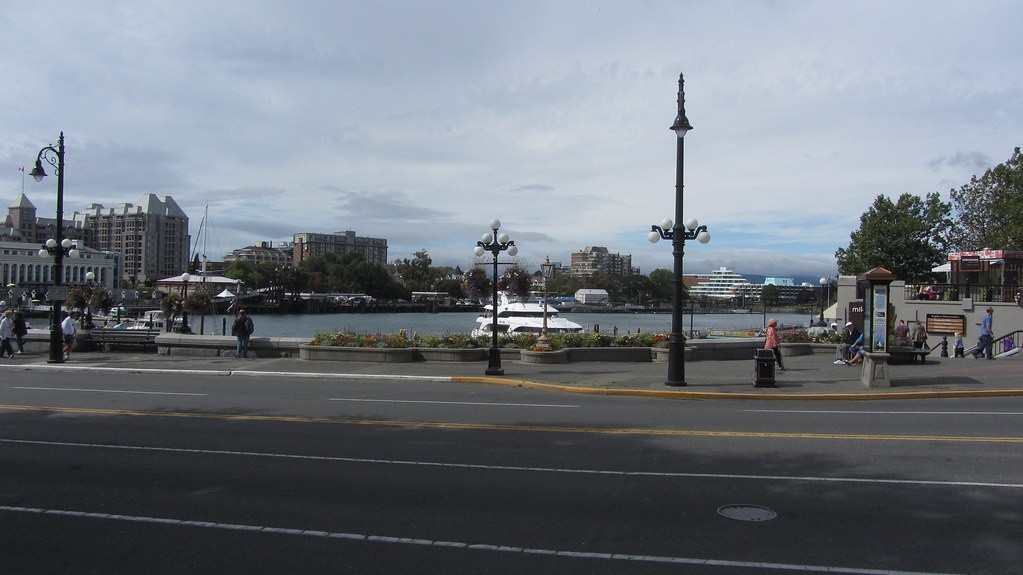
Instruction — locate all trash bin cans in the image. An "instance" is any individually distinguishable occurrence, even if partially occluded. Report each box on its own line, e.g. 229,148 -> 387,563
753,349 -> 777,388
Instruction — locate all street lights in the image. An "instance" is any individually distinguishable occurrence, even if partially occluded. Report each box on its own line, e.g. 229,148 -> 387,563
86,271 -> 96,325
29,131 -> 80,364
180,273 -> 191,332
817,278 -> 827,327
474,220 -> 518,377
648,71 -> 711,387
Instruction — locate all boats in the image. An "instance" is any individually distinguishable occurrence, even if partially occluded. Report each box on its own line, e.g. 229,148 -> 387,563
471,289 -> 584,337
126,310 -> 184,333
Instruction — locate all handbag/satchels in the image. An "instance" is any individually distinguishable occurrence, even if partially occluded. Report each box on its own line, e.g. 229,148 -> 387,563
245,319 -> 253,334
64,334 -> 73,344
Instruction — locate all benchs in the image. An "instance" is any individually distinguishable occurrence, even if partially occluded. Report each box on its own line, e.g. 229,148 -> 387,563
88,327 -> 161,353
888,347 -> 931,364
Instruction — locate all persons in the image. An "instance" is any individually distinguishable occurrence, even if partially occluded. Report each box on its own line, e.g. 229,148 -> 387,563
232,310 -> 251,358
75,315 -> 82,331
63,311 -> 77,361
953,332 -> 965,359
834,322 -> 864,365
915,280 -> 944,300
1000,334 -> 1015,352
894,319 -> 928,349
972,307 -> 997,360
765,319 -> 788,371
0,310 -> 28,360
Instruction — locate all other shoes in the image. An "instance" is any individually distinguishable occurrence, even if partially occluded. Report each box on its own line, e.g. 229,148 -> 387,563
9,354 -> 14,359
971,352 -> 977,359
834,359 -> 843,365
65,356 -> 68,360
845,360 -> 851,366
17,350 -> 23,354
986,356 -> 996,360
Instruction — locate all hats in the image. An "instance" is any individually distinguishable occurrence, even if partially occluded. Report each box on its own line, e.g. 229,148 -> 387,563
768,319 -> 779,326
845,322 -> 852,326
239,310 -> 245,313
